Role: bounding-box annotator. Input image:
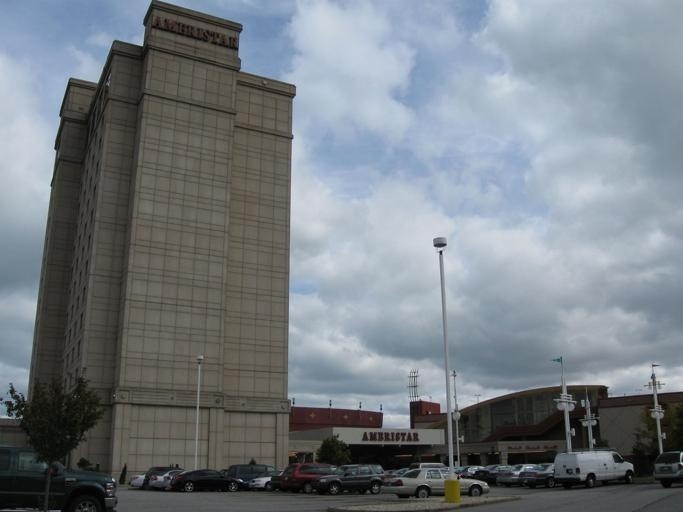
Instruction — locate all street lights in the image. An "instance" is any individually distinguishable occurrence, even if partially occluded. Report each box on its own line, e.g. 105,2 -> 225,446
194,356 -> 203,471
432,237 -> 460,502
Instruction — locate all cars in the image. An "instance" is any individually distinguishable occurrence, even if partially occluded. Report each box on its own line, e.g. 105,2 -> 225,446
653,452 -> 683,487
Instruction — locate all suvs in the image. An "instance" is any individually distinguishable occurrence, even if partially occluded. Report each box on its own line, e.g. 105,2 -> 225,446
1,447 -> 118,512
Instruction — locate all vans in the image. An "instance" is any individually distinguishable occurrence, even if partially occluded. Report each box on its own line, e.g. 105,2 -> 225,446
552,450 -> 635,488
